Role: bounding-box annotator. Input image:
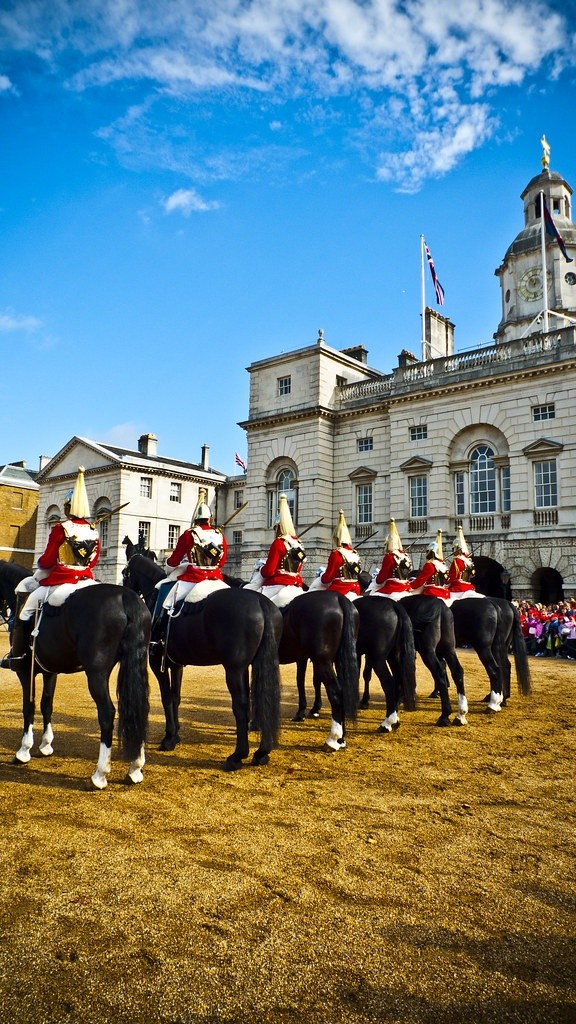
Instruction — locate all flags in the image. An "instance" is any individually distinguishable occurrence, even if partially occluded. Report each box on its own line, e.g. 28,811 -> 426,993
543,193 -> 574,263
235,453 -> 247,474
424,240 -> 444,306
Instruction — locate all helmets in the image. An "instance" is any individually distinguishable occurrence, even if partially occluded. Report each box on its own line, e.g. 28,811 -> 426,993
272,494 -> 297,536
426,529 -> 444,560
190,490 -> 210,527
63,466 -> 91,518
453,526 -> 469,553
384,518 -> 403,552
331,509 -> 352,547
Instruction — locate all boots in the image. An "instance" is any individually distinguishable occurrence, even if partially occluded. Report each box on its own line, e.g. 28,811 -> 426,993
150,607 -> 169,643
7,618 -> 28,660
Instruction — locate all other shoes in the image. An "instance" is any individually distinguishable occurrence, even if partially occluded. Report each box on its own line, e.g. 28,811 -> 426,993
540,653 -> 544,657
535,653 -> 540,657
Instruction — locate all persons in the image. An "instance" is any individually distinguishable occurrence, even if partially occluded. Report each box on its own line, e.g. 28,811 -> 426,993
317,509 -> 360,596
365,518 -> 413,598
444,525 -> 475,592
408,529 -> 451,601
150,488 -> 228,655
254,494 -> 308,599
510,597 -> 576,660
0,465 -> 101,669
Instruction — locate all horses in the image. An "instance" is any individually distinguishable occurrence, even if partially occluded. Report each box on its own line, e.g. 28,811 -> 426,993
124,554 -> 284,769
222,574 -> 359,752
442,595 -> 533,713
301,584 -> 417,733
122,535 -> 157,562
0,558 -> 152,789
360,591 -> 469,727
